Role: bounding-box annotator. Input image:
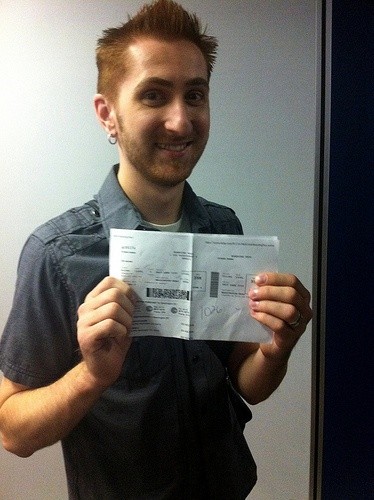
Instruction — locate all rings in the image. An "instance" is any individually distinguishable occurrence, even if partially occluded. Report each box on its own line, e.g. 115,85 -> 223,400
288,312 -> 304,328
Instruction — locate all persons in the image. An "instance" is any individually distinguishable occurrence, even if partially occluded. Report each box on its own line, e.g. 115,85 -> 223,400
0,1 -> 312,500
